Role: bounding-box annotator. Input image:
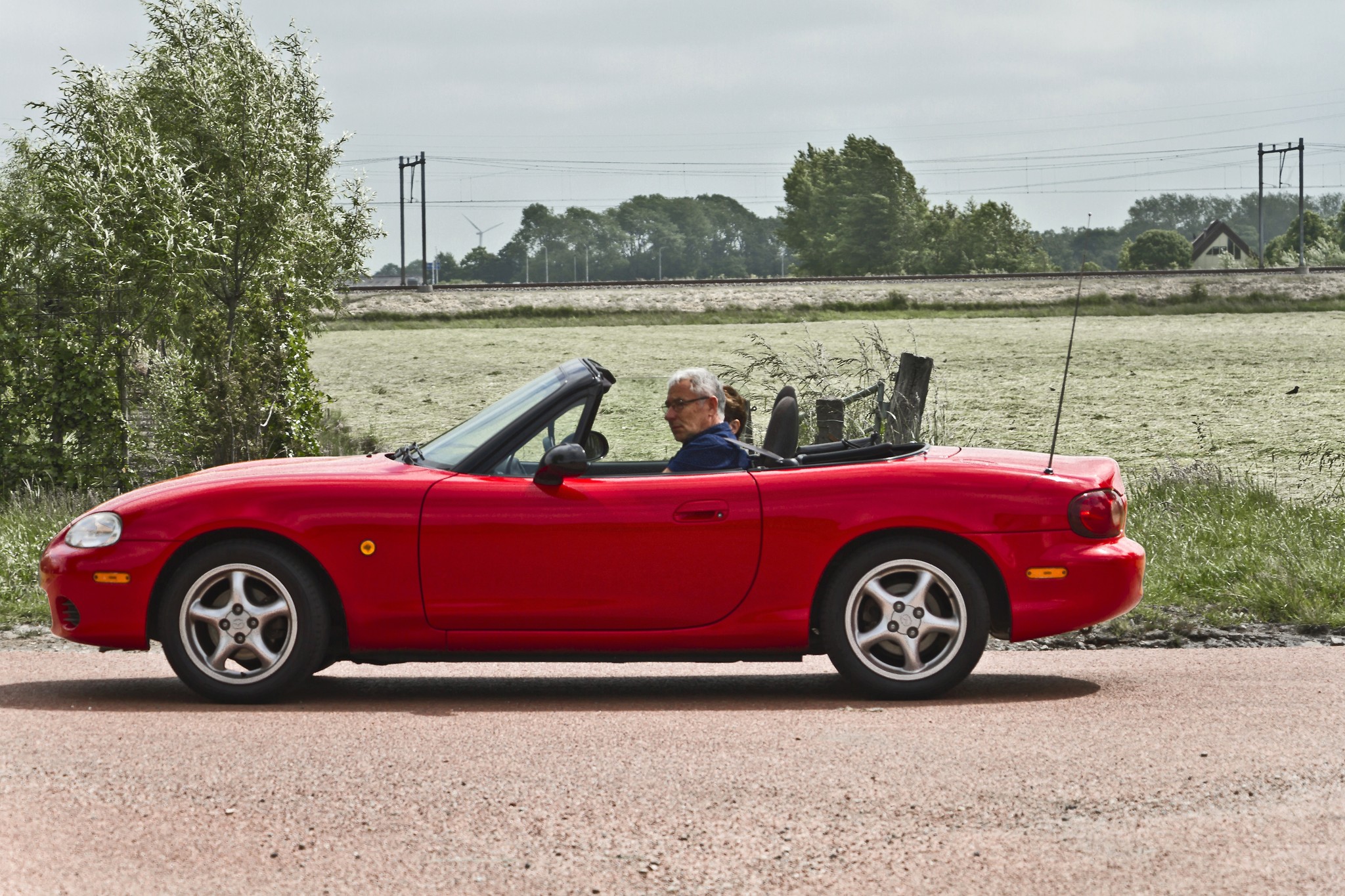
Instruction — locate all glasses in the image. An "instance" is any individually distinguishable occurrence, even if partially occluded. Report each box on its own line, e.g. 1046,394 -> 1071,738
661,395 -> 711,413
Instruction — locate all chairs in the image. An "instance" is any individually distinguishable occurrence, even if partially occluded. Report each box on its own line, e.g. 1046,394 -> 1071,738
758,386 -> 800,470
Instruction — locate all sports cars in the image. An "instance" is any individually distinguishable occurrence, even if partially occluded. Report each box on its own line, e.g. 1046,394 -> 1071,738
37,349 -> 1146,703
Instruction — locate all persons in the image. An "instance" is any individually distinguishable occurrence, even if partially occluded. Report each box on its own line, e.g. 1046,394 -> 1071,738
722,384 -> 749,455
660,366 -> 753,473
